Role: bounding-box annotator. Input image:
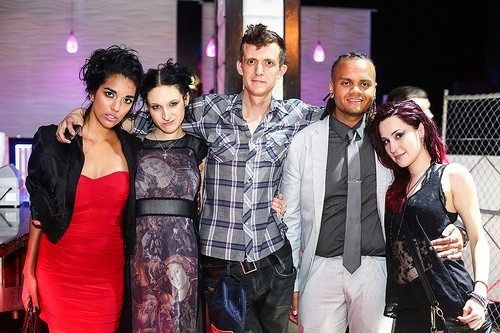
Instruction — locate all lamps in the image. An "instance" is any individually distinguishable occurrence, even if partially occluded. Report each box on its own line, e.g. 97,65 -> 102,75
66,0 -> 78,53
313,0 -> 326,62
206,34 -> 216,57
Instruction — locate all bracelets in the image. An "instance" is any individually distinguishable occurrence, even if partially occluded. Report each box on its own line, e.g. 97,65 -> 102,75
474,281 -> 489,289
470,292 -> 487,308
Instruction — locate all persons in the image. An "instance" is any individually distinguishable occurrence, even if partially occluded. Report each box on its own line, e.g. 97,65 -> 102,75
368,99 -> 492,333
20,45 -> 140,333
131,58 -> 287,333
53,23 -> 327,333
388,86 -> 435,124
282,50 -> 470,333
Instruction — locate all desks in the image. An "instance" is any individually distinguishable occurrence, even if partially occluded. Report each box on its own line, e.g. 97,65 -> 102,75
0,205 -> 34,314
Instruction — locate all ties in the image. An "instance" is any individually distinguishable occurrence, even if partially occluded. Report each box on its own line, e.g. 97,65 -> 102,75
342,130 -> 362,274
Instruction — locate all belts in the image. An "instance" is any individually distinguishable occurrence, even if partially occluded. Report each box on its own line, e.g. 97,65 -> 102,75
203,243 -> 292,276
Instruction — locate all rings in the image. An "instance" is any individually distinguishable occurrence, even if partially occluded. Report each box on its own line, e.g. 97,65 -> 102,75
449,235 -> 453,243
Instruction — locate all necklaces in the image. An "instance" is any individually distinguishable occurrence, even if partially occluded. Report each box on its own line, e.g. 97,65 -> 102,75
152,131 -> 179,158
402,170 -> 423,202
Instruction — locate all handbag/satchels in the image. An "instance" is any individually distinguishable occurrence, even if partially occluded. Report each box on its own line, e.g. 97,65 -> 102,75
428,306 -> 492,333
